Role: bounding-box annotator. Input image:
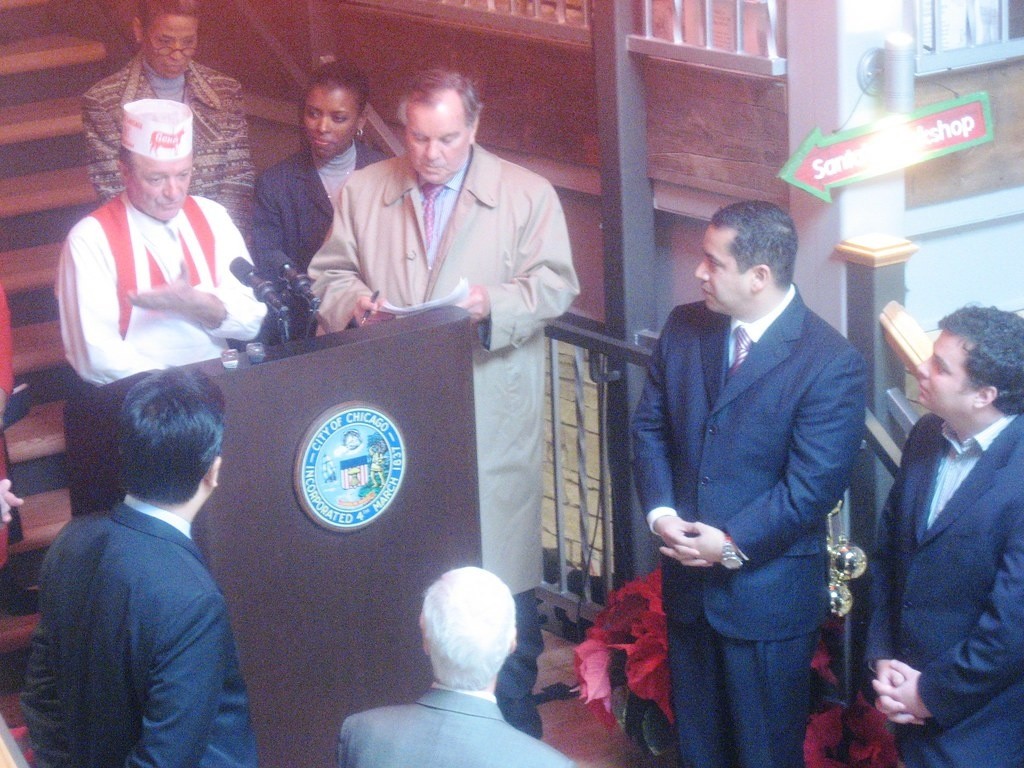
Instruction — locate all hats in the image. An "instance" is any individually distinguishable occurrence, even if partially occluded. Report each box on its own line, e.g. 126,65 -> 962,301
120,99 -> 194,162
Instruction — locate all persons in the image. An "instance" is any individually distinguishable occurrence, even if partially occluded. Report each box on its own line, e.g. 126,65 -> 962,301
79,0 -> 255,250
253,59 -> 391,346
20,366 -> 259,768
0,281 -> 26,571
864,305 -> 1024,768
336,566 -> 580,768
53,98 -> 269,387
631,201 -> 867,768
307,70 -> 580,741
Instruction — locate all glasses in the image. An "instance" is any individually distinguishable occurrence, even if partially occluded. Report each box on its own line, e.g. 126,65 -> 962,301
144,25 -> 199,58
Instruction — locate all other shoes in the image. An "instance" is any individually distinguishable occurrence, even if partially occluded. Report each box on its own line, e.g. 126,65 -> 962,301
504,692 -> 542,737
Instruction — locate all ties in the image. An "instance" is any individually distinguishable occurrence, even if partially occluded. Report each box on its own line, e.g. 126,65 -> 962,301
421,182 -> 445,253
728,327 -> 753,380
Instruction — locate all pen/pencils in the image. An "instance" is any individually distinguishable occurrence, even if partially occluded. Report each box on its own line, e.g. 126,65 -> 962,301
360,288 -> 380,327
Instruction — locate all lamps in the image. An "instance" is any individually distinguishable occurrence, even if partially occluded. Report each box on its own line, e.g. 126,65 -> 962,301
857,30 -> 917,114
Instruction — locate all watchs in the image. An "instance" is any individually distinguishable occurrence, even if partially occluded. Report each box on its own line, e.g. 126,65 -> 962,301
720,535 -> 744,570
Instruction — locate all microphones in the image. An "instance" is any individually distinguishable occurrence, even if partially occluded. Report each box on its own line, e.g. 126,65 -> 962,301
272,252 -> 323,306
228,255 -> 290,313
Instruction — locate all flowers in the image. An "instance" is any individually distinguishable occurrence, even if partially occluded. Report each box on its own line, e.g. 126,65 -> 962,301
803,690 -> 899,768
569,562 -> 838,768
